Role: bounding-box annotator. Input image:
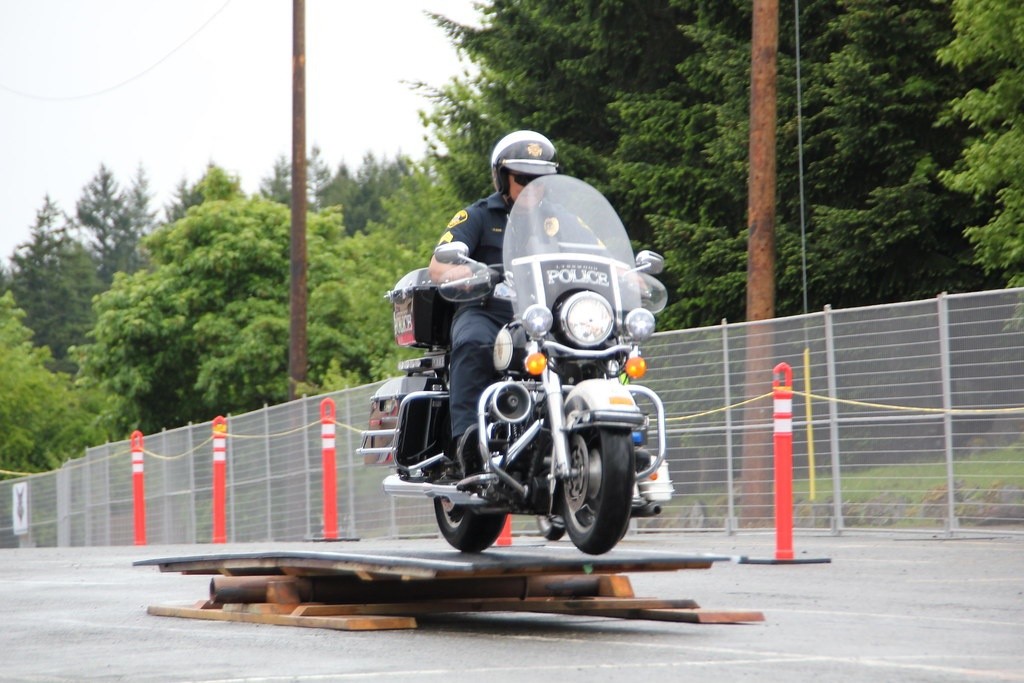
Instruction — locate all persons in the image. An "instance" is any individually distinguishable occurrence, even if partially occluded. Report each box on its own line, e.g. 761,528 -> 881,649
429,129 -> 650,479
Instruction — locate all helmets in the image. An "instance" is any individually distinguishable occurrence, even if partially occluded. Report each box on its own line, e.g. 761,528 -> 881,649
490,129 -> 560,210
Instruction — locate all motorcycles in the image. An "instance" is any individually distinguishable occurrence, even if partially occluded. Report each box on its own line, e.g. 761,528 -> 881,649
354,170 -> 676,553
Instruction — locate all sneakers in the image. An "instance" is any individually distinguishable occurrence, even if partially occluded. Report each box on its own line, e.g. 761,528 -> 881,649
634,446 -> 651,467
455,423 -> 479,459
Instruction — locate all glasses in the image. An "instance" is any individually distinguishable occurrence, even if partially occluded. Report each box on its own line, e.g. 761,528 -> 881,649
507,172 -> 538,186
